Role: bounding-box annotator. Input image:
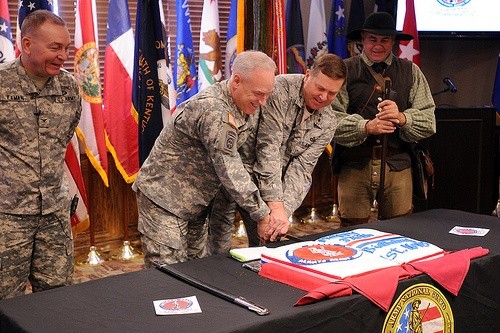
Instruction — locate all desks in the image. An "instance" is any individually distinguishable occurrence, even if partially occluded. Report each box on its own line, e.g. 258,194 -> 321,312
0,209 -> 500,333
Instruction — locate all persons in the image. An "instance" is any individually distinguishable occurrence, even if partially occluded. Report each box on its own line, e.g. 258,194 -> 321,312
132,50 -> 277,270
206,53 -> 348,257
331,12 -> 437,228
0,10 -> 83,301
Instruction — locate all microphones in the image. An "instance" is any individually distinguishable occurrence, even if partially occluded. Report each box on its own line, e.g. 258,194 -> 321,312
443,77 -> 458,92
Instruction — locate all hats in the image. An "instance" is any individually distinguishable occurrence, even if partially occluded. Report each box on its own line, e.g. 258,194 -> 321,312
345,12 -> 414,41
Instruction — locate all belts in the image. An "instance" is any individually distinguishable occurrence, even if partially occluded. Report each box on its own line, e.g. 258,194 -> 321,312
366,144 -> 404,160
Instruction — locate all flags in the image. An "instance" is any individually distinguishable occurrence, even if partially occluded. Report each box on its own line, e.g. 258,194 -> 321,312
225,0 -> 243,79
73,0 -> 108,188
0,0 -> 17,70
132,0 -> 177,170
173,0 -> 198,106
396,0 -> 421,66
197,0 -> 222,93
286,0 -> 398,74
103,0 -> 139,184
15,0 -> 91,239
